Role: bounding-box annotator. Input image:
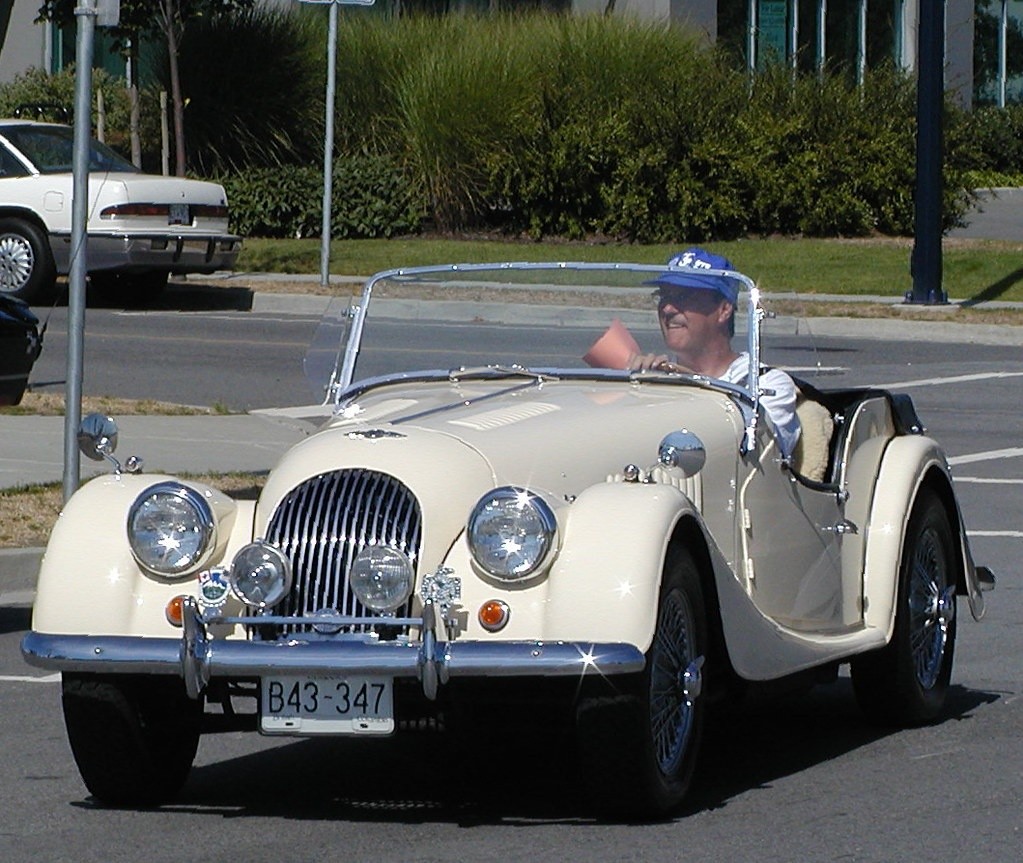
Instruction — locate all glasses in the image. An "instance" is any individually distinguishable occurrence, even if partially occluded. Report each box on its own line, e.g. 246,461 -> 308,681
650,289 -> 721,306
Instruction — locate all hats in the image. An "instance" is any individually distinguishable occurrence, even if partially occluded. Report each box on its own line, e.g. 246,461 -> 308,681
640,247 -> 740,308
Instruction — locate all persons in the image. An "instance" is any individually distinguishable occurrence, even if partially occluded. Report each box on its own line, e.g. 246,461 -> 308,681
628,251 -> 803,466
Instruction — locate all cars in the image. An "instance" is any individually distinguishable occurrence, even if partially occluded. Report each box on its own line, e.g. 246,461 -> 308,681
0,118 -> 245,310
18,262 -> 996,826
